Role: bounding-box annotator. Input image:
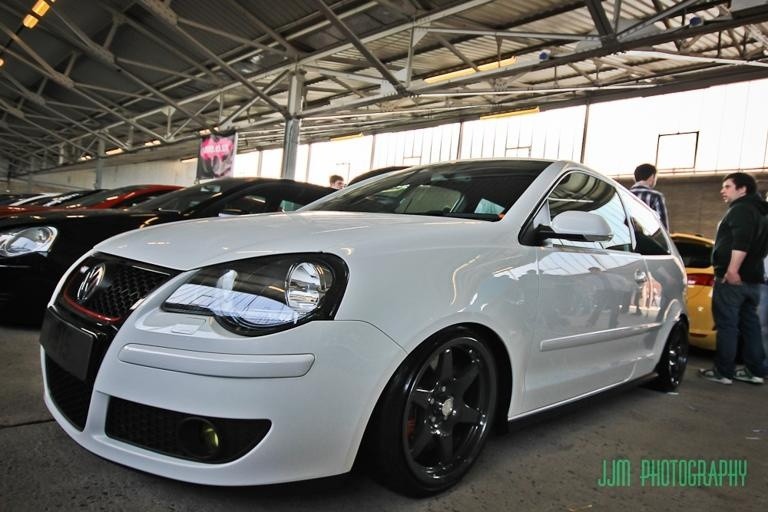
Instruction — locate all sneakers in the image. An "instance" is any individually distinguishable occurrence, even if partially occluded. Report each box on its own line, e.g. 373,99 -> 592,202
733,367 -> 764,383
698,368 -> 732,385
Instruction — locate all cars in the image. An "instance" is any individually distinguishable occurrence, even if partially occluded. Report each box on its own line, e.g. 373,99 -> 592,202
0,177 -> 390,325
35,153 -> 693,502
0,177 -> 284,220
668,228 -> 717,353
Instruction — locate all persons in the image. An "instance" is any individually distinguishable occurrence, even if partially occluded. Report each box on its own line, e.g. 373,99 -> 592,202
697,173 -> 768,386
629,164 -> 669,235
330,174 -> 345,190
